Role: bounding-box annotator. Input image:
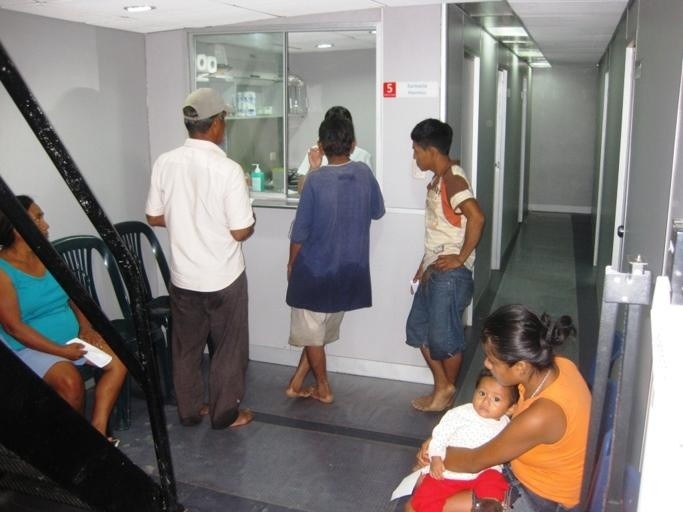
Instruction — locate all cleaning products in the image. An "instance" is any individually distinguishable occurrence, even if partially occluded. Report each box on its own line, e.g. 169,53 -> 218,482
250,162 -> 264,192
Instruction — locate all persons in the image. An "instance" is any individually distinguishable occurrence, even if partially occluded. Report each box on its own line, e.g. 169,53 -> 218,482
407,370 -> 518,511
285,115 -> 384,403
1,195 -> 128,449
296,105 -> 370,196
405,120 -> 486,412
404,303 -> 595,512
146,88 -> 256,430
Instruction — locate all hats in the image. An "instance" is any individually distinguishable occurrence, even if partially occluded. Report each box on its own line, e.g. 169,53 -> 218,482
181,87 -> 233,121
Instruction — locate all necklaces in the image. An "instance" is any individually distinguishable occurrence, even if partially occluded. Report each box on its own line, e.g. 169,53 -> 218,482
530,368 -> 552,400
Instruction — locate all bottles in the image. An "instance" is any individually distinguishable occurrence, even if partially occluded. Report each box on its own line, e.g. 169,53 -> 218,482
272,167 -> 283,193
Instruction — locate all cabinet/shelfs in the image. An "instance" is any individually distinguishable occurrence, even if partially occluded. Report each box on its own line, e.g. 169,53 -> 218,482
196,78 -> 305,121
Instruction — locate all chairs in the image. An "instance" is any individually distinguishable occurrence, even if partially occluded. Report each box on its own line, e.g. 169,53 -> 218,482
49,234 -> 161,432
584,329 -> 626,510
103,220 -> 213,405
